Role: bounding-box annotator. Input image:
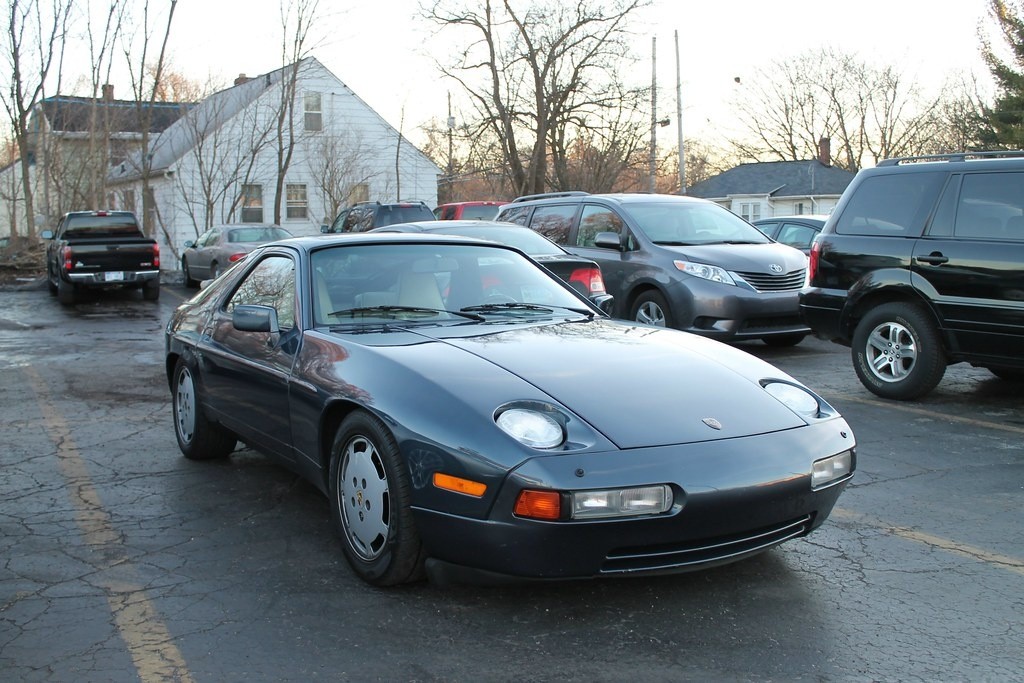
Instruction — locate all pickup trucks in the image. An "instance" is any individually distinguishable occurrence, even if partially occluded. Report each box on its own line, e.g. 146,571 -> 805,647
40,209 -> 161,304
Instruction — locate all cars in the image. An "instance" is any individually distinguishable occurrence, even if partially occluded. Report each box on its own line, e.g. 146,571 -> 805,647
180,223 -> 296,287
310,220 -> 614,324
743,214 -> 905,256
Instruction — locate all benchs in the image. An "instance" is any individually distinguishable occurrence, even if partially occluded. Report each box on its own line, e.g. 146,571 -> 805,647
333,285 -> 401,323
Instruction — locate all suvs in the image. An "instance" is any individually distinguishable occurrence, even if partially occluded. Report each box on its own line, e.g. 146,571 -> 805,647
320,200 -> 513,234
797,149 -> 1024,402
493,190 -> 813,347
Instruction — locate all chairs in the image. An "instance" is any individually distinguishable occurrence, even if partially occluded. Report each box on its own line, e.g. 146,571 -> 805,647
392,268 -> 452,321
278,268 -> 345,328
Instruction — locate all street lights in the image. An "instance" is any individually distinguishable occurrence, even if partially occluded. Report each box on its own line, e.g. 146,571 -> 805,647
649,118 -> 671,194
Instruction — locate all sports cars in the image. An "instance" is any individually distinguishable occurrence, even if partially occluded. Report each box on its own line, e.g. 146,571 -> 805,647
164,235 -> 857,587
